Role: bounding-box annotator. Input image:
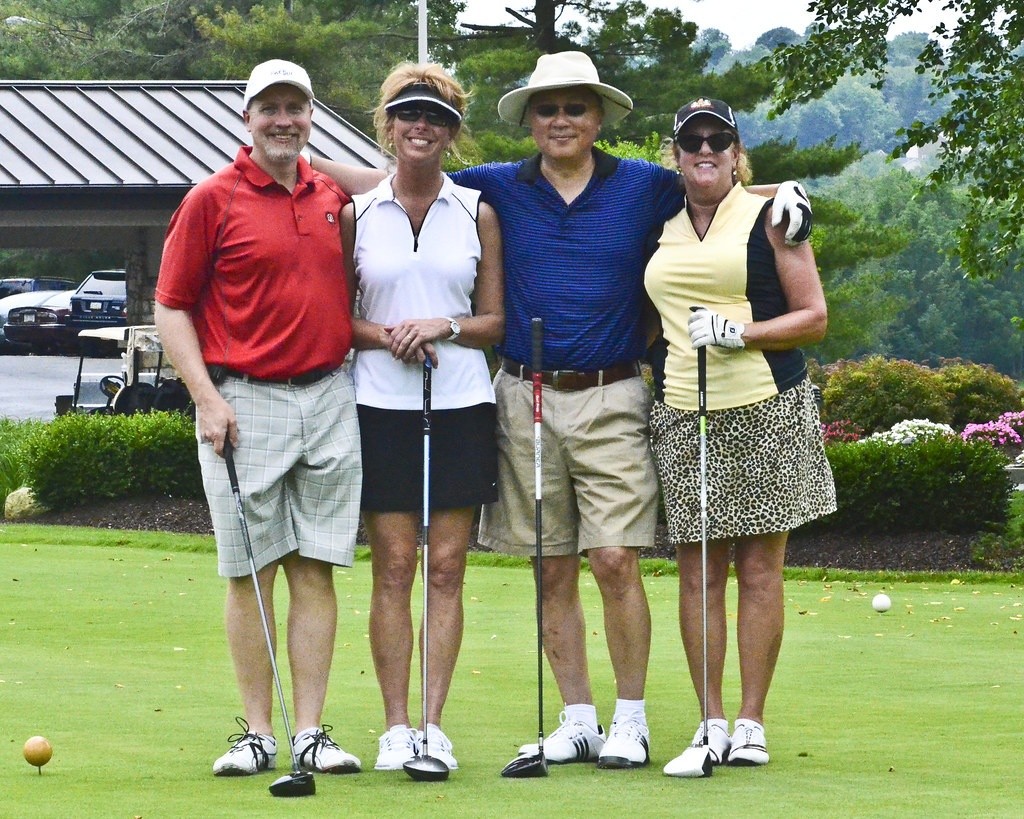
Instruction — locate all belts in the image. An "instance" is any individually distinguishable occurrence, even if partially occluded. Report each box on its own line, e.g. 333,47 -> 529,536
500,355 -> 641,392
224,365 -> 339,386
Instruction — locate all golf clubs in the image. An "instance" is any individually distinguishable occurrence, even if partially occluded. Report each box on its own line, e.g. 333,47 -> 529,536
402,349 -> 454,783
221,429 -> 317,799
661,303 -> 716,780
500,315 -> 551,781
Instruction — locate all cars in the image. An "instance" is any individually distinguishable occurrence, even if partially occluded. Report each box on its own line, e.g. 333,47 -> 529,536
0,274 -> 80,355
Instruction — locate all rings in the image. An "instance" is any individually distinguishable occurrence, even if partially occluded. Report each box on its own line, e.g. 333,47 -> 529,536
407,335 -> 412,341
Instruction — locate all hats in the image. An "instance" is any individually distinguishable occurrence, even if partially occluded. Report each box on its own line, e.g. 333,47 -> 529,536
498,49 -> 633,125
384,82 -> 462,124
672,97 -> 734,135
243,58 -> 314,112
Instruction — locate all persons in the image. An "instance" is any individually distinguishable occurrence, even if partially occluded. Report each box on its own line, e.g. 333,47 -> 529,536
644,98 -> 837,764
336,61 -> 503,771
153,60 -> 363,777
300,51 -> 814,768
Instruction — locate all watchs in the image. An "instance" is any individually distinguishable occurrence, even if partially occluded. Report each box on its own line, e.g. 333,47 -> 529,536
446,317 -> 460,340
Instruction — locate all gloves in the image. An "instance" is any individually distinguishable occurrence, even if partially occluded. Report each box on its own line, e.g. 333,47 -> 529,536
772,180 -> 813,247
687,304 -> 746,351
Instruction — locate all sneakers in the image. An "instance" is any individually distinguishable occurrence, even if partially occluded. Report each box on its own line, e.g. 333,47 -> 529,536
598,709 -> 649,769
374,724 -> 416,770
544,702 -> 607,763
213,716 -> 277,776
416,722 -> 459,770
690,723 -> 731,765
727,725 -> 769,764
291,724 -> 362,772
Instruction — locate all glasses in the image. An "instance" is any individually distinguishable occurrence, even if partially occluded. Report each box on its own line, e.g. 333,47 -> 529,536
674,132 -> 736,153
395,107 -> 448,127
536,103 -> 585,117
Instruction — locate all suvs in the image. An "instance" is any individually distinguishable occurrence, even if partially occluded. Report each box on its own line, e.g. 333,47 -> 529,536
65,268 -> 128,354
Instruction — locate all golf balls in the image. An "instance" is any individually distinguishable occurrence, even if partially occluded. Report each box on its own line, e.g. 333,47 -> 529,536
22,735 -> 53,767
871,593 -> 892,613
